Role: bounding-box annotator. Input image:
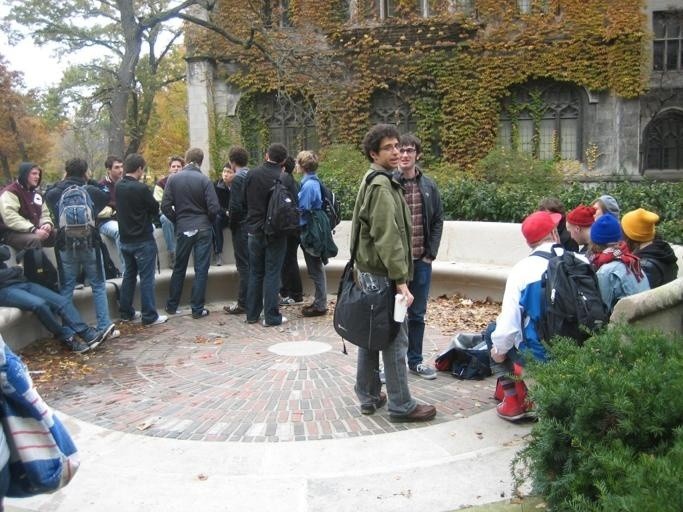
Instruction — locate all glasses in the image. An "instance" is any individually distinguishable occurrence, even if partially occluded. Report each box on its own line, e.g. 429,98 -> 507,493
398,148 -> 417,153
379,143 -> 400,151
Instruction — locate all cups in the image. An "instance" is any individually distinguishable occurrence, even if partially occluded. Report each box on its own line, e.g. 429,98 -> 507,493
393,294 -> 407,322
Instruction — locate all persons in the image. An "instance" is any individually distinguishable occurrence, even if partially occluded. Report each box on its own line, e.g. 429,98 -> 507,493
366,132 -> 441,383
0,236 -> 115,354
2,141 -> 331,328
484,194 -> 679,421
349,124 -> 436,425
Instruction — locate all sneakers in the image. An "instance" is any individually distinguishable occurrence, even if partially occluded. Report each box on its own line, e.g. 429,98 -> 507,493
514,377 -> 543,419
495,393 -> 522,422
63,304 -> 177,353
360,389 -> 387,415
388,400 -> 437,424
407,360 -> 439,381
192,308 -> 210,320
223,295 -> 327,327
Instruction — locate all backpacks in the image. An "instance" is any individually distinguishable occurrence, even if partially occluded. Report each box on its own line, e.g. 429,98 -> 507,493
303,176 -> 342,233
264,178 -> 303,243
533,254 -> 611,347
56,182 -> 98,254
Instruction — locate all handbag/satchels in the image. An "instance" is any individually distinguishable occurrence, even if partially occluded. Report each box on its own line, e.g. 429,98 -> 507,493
1,331 -> 82,498
331,260 -> 404,353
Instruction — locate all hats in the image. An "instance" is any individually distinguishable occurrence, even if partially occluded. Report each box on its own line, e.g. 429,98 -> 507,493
521,194 -> 659,244
